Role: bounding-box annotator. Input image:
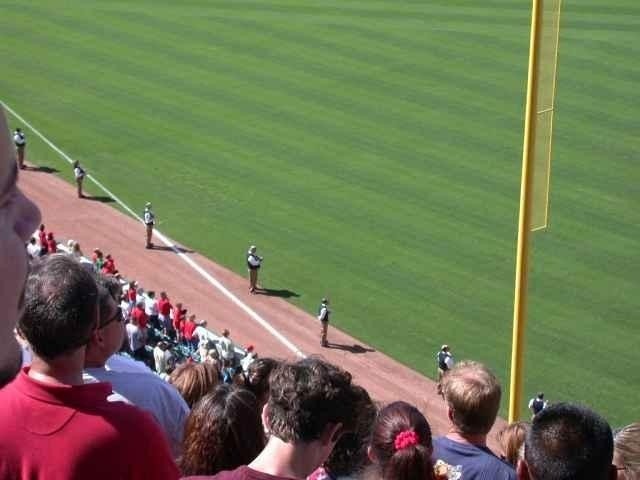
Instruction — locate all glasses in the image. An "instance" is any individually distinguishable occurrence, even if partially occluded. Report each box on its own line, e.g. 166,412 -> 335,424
99,307 -> 124,330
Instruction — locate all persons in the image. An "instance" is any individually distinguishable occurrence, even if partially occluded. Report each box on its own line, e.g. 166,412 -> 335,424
432,360 -> 517,480
497,420 -> 531,467
516,398 -> 617,480
144,201 -> 156,249
437,344 -> 453,394
612,422 -> 640,480
528,391 -> 549,419
72,159 -> 86,198
1,104 -> 44,392
2,225 -> 433,480
483,414 -> 508,459
14,127 -> 26,169
